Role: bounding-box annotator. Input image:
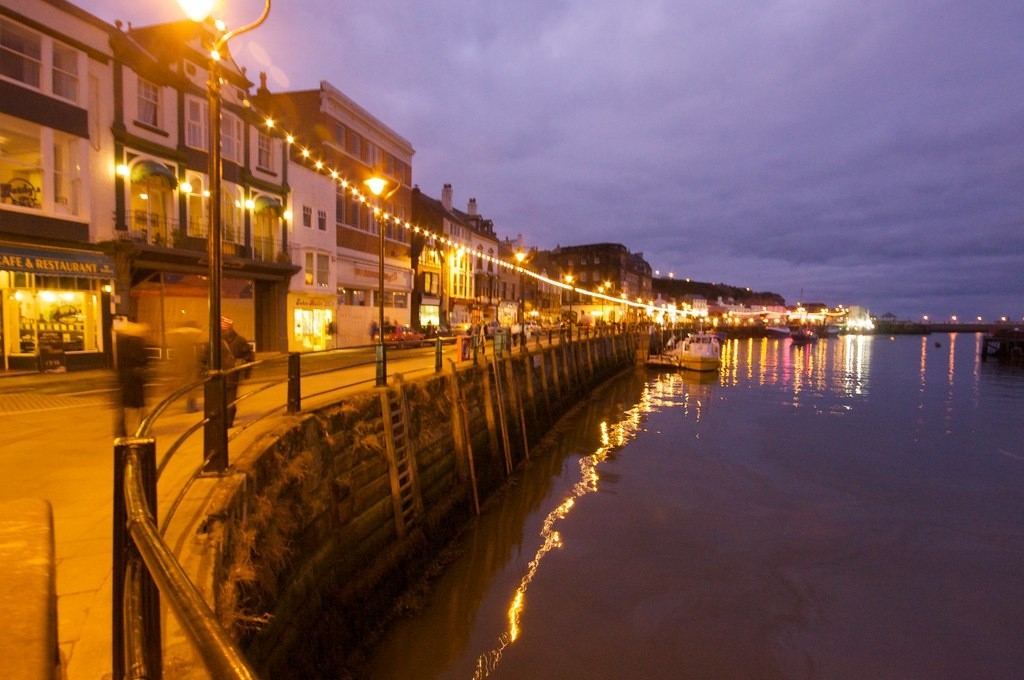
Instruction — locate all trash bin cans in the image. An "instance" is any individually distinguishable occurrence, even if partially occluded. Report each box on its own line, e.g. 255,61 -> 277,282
493,327 -> 512,357
456,335 -> 471,361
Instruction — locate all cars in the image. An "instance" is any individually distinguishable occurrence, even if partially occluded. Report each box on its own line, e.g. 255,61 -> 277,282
485,320 -> 505,339
413,324 -> 458,345
521,319 -> 595,337
373,324 -> 426,348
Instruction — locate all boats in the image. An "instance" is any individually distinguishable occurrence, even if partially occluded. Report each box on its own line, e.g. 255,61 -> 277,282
819,325 -> 840,338
763,324 -> 792,337
789,323 -> 819,344
676,331 -> 723,371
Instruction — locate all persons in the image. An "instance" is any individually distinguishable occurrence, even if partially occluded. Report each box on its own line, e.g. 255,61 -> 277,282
370,317 -> 436,339
199,314 -> 256,428
467,318 -> 528,347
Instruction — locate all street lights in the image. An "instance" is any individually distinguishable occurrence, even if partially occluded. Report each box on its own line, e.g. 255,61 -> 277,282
511,240 -> 535,349
186,1 -> 275,474
363,165 -> 406,386
597,286 -> 605,329
561,270 -> 577,337
429,231 -> 465,372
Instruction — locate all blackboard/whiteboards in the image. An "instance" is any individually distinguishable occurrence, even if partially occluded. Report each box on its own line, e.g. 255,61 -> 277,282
38,330 -> 67,374
